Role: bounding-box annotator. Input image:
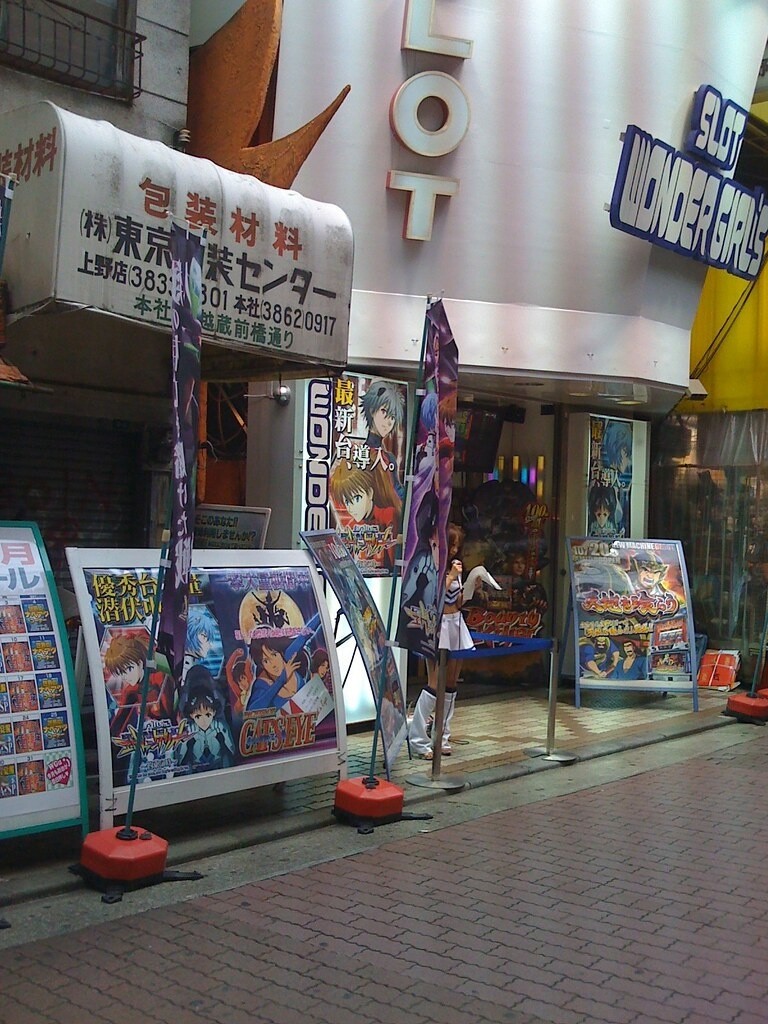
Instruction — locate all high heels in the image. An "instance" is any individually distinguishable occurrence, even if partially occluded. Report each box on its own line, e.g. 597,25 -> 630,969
418,752 -> 434,760
441,748 -> 452,756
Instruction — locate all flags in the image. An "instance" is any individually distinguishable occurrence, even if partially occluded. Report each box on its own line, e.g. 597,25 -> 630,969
157,225 -> 204,688
394,298 -> 460,664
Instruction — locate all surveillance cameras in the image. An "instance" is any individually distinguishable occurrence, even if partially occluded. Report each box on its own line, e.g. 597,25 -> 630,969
685,379 -> 708,401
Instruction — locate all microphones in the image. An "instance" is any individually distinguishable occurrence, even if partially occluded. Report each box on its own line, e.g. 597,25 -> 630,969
451,559 -> 464,592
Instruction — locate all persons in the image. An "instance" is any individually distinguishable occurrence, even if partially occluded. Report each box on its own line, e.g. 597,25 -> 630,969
407,523 -> 483,760
